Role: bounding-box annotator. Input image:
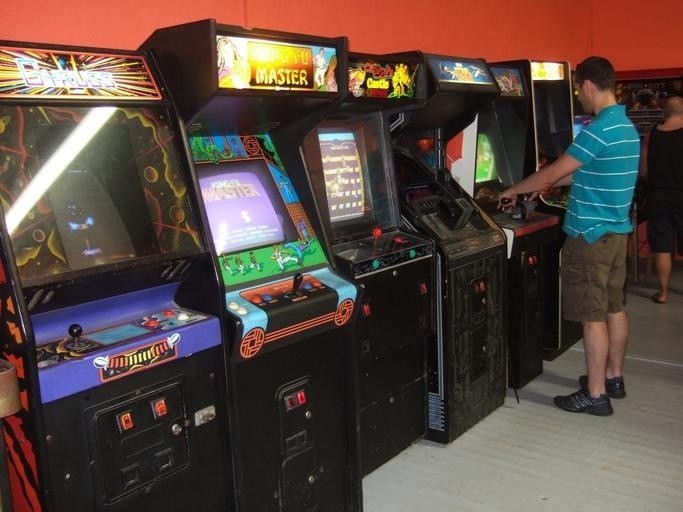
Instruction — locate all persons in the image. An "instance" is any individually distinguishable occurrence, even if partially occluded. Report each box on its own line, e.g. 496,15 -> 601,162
640,95 -> 682,305
495,55 -> 641,418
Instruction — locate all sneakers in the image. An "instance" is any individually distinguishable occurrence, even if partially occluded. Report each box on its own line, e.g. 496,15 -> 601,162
579,376 -> 626,398
554,386 -> 613,416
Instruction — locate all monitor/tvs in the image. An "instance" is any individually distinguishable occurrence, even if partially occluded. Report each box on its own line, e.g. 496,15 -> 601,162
476,131 -> 496,186
573,115 -> 592,142
188,131 -> 328,286
392,148 -> 435,186
316,125 -> 378,227
0,105 -> 201,281
